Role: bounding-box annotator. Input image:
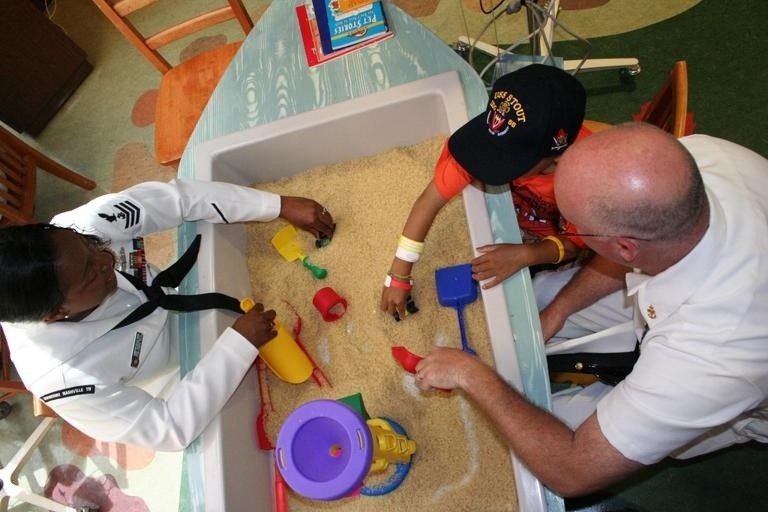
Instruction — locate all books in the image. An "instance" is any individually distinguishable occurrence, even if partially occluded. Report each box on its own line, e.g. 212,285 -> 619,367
295,0 -> 395,67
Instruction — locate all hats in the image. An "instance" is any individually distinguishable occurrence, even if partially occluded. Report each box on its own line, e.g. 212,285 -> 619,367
447,64 -> 588,187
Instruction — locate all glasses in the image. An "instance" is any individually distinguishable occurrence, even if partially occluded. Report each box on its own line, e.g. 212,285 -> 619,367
557,215 -> 651,243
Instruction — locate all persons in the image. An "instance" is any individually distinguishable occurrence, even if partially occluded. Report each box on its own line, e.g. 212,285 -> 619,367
415,116 -> 768,511
0,177 -> 336,456
379,60 -> 630,328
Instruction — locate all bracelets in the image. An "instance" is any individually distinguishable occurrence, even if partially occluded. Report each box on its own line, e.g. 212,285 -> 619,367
386,269 -> 411,282
542,233 -> 566,267
382,273 -> 415,292
395,245 -> 419,265
398,236 -> 427,255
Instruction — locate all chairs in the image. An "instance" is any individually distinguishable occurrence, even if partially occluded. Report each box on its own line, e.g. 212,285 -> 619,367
93,1 -> 256,175
1,123 -> 96,226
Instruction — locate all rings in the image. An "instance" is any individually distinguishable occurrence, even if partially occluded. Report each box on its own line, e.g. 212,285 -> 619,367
321,206 -> 329,214
